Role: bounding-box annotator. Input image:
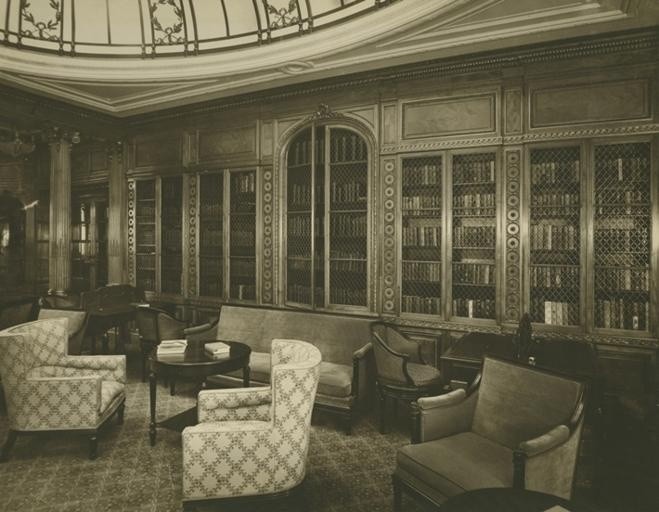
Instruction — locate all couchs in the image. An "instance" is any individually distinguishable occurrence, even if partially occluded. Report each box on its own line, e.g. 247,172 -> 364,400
180,302 -> 378,436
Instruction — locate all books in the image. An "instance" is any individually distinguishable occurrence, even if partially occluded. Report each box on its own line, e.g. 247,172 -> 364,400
452,154 -> 496,319
594,154 -> 651,331
402,156 -> 441,316
288,134 -> 368,307
530,154 -> 580,327
156,338 -> 188,356
135,172 -> 255,303
204,341 -> 232,356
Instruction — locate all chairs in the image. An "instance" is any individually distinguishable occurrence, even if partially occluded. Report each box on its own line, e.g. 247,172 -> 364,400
366,318 -> 440,431
391,357 -> 592,512
1,317 -> 127,460
186,335 -> 323,512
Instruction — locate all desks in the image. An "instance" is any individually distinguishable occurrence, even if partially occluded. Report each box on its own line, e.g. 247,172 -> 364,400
146,339 -> 251,448
432,486 -> 583,512
438,330 -> 596,394
